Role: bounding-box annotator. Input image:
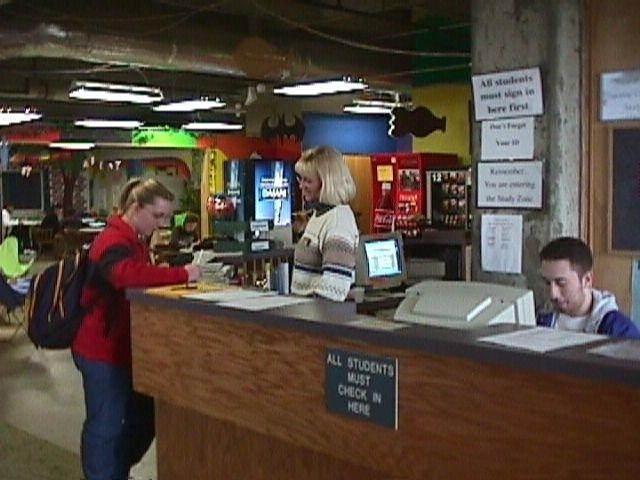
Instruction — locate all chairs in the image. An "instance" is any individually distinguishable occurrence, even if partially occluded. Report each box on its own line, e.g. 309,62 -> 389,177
0,226 -> 82,345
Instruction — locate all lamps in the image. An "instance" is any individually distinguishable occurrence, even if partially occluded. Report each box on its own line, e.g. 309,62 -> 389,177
69,80 -> 164,106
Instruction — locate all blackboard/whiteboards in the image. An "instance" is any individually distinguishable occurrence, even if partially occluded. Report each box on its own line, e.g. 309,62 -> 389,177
606,121 -> 640,256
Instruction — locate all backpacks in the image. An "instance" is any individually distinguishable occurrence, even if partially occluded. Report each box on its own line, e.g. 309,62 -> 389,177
23,240 -> 128,349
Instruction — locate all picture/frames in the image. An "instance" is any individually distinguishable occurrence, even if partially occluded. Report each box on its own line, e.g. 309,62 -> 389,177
471,64 -> 546,123
473,157 -> 548,212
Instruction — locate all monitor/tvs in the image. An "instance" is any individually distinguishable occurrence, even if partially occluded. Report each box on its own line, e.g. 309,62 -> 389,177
349,232 -> 407,303
394,281 -> 535,330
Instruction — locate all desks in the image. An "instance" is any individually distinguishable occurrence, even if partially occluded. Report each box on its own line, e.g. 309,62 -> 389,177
78,227 -> 103,248
163,251 -> 192,268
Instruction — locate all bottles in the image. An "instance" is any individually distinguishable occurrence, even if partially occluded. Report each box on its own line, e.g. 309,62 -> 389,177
374,182 -> 393,234
431,172 -> 466,230
397,170 -> 421,238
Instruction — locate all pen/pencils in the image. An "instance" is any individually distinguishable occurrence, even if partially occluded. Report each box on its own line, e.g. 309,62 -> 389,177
196,249 -> 204,265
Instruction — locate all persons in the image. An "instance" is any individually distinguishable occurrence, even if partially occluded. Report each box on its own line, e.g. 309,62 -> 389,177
167,215 -> 198,252
2,201 -> 19,239
41,205 -> 61,233
288,141 -> 359,304
536,237 -> 638,345
401,170 -> 421,189
60,208 -> 82,231
73,175 -> 203,480
